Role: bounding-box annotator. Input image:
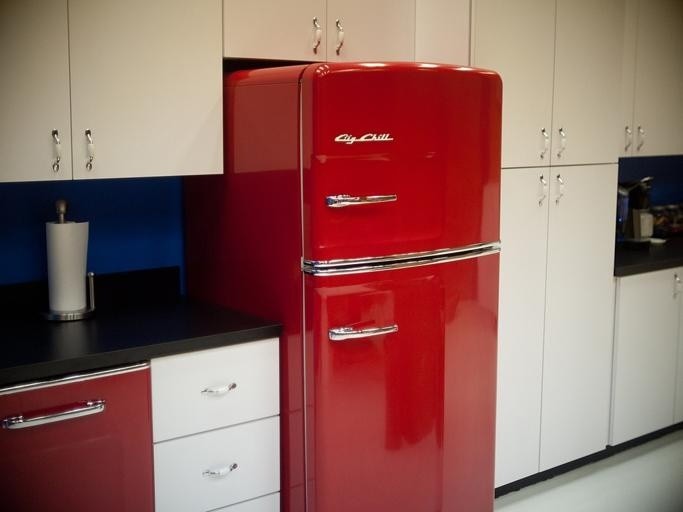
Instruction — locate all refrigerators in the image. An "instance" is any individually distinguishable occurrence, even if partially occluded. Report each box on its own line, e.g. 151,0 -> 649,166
181,59 -> 503,510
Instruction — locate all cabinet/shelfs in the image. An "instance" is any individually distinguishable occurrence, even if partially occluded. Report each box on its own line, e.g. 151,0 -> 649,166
494,164 -> 617,499
2,2 -> 223,182
151,336 -> 280,512
469,1 -> 618,168
619,2 -> 683,158
222,1 -> 415,68
607,266 -> 682,458
4,360 -> 155,508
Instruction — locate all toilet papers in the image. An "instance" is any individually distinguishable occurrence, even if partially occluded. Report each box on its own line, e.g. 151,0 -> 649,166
44,218 -> 91,313
